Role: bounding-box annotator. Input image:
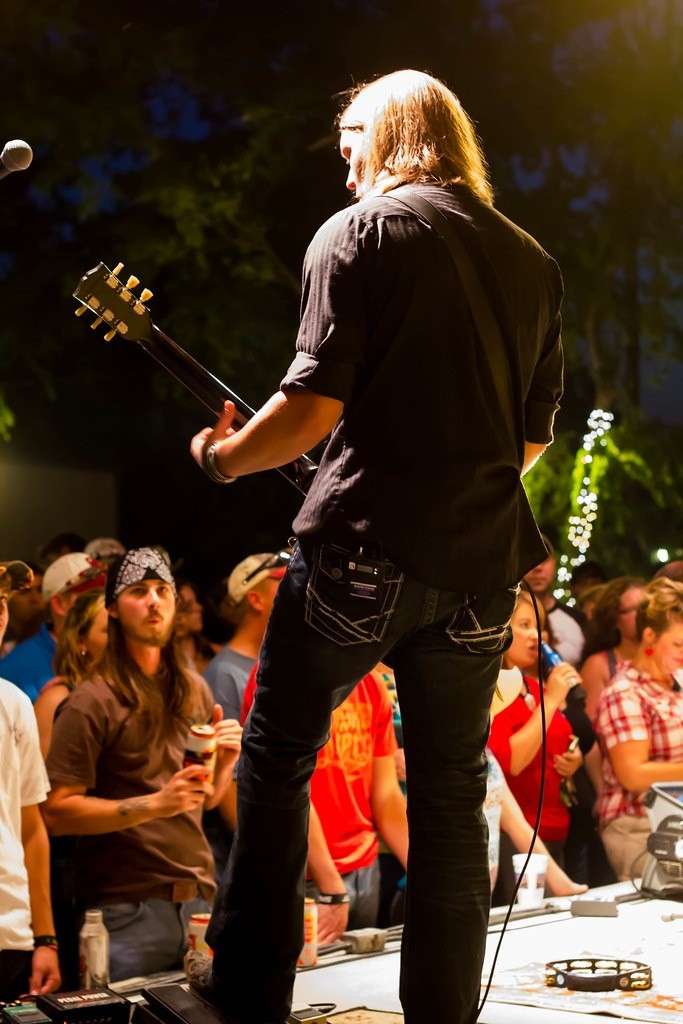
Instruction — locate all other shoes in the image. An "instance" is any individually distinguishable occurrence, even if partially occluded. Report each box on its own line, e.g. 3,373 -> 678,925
190,968 -> 224,1014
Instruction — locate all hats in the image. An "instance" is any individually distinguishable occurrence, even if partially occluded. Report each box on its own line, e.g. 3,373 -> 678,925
43,554 -> 107,599
226,554 -> 287,606
0,560 -> 34,591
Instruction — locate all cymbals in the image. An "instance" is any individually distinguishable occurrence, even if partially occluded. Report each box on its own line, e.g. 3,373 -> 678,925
541,956 -> 654,992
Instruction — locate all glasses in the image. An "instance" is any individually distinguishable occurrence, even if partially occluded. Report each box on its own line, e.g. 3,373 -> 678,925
243,550 -> 290,583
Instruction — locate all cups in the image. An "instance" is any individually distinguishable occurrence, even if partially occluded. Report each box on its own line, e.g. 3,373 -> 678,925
512,852 -> 549,907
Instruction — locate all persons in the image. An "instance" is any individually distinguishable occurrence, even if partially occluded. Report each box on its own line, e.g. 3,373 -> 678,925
183,70 -> 566,1024
0,531 -> 683,1001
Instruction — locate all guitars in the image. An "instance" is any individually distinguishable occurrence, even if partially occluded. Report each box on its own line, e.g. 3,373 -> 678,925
70,264 -> 318,495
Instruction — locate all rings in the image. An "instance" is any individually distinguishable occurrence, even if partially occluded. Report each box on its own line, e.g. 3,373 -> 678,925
567,677 -> 576,686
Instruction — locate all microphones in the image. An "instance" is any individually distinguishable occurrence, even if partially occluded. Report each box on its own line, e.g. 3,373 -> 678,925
0,139 -> 33,179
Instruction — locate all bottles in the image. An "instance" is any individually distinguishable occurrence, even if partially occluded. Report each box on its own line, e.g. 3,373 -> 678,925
385,877 -> 406,927
539,640 -> 587,704
78,909 -> 110,992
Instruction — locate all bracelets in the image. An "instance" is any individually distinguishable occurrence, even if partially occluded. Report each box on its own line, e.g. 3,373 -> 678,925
206,442 -> 237,483
318,893 -> 351,904
34,935 -> 58,950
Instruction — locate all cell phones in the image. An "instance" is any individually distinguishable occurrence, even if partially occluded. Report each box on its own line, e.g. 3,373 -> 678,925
565,738 -> 579,762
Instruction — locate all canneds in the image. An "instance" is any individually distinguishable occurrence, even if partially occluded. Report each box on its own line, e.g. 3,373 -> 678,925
182,723 -> 216,788
297,896 -> 319,968
187,913 -> 213,959
